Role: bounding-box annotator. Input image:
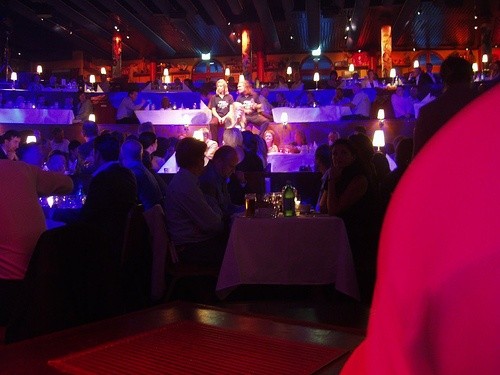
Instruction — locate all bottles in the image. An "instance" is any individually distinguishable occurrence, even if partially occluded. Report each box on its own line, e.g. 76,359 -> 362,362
283,180 -> 295,217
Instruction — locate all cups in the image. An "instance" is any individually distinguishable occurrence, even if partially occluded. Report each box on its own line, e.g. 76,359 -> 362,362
245,194 -> 257,217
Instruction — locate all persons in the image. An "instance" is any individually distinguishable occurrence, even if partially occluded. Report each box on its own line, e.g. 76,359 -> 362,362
0,55 -> 500,375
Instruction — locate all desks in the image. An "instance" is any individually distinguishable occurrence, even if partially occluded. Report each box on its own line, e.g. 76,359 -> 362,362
155,152 -> 178,173
226,204 -> 344,300
267,153 -> 316,171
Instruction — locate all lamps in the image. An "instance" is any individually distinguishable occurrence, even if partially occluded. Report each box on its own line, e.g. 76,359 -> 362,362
313,72 -> 319,91
372,129 -> 386,152
89,74 -> 96,91
377,109 -> 385,126
312,45 -> 321,63
388,69 -> 396,87
482,54 -> 488,69
349,64 -> 355,78
163,68 -> 171,85
182,115 -> 191,134
10,72 -> 17,84
37,65 -> 42,75
224,68 -> 231,82
280,112 -> 288,129
472,63 -> 478,79
286,66 -> 293,83
